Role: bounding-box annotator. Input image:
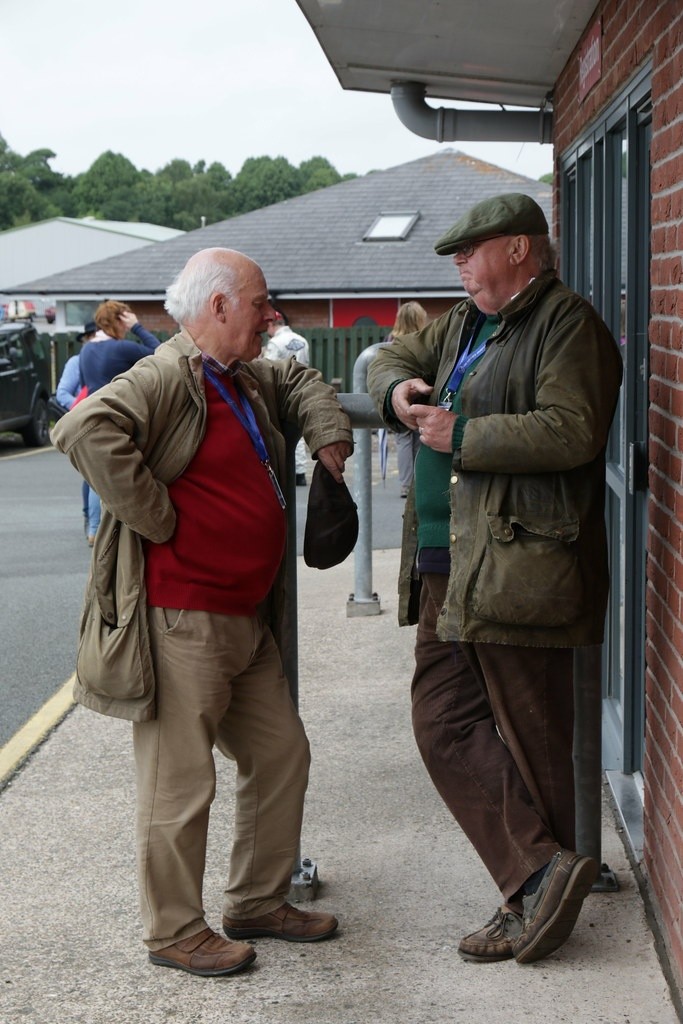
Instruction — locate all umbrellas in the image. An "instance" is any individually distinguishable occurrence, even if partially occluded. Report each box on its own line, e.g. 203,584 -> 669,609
379,428 -> 388,487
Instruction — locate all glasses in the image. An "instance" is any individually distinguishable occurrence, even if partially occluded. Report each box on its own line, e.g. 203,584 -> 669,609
455,233 -> 509,258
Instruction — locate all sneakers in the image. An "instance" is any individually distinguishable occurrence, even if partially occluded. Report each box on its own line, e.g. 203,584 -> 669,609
221,899 -> 339,944
456,904 -> 524,963
512,850 -> 598,963
146,926 -> 259,978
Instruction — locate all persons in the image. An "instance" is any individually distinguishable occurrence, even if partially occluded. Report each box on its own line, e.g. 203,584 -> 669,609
387,302 -> 426,497
367,194 -> 625,963
57,301 -> 160,546
255,315 -> 309,368
49,246 -> 354,976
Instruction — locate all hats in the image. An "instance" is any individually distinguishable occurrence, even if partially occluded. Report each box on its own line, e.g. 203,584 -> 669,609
75,320 -> 99,344
434,192 -> 551,257
303,459 -> 360,571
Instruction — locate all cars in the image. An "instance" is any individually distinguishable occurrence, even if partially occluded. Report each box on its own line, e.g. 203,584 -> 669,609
0,323 -> 50,447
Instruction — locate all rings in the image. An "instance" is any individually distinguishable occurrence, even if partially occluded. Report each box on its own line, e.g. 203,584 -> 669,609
419,427 -> 423,434
